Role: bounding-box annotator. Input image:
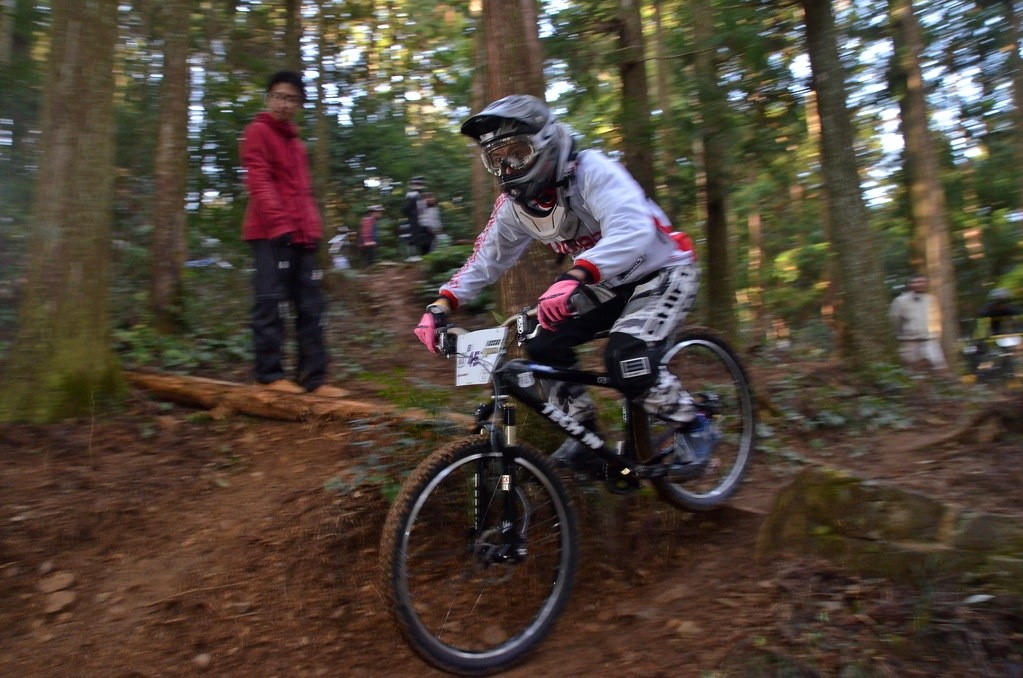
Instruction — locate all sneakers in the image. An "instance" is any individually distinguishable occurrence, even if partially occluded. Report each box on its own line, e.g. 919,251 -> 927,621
551,438 -> 594,470
669,414 -> 722,483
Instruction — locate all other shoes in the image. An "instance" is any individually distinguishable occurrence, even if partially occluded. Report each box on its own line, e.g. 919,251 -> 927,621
254,377 -> 307,394
311,385 -> 351,397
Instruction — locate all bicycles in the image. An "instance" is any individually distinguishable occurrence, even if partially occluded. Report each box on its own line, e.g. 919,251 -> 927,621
376,289 -> 760,678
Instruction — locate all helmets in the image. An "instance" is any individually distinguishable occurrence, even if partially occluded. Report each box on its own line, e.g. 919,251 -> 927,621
460,93 -> 561,205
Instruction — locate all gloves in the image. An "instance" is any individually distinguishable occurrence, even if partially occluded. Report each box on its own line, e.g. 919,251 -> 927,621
538,273 -> 582,333
414,304 -> 454,356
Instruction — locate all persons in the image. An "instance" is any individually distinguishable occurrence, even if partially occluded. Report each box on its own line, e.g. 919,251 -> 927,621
889,274 -> 948,373
238,71 -> 357,400
414,95 -> 721,485
328,176 -> 442,269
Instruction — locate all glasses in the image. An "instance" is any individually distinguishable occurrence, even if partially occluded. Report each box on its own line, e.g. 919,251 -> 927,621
481,135 -> 535,176
